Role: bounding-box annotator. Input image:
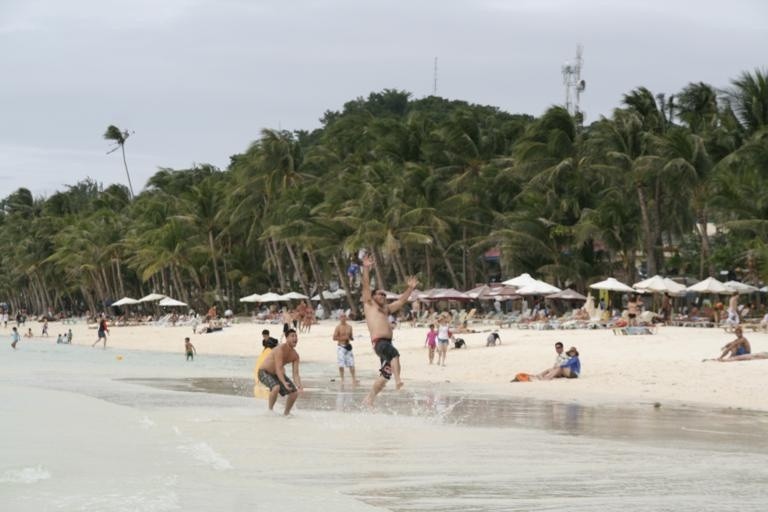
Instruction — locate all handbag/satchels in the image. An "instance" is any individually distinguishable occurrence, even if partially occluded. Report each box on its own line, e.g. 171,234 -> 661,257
344,344 -> 351,350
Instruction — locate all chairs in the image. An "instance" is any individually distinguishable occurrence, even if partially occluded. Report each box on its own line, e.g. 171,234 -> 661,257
403,301 -> 759,333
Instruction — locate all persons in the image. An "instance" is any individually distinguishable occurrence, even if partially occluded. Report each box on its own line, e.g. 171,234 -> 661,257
426,320 -> 466,366
2,307 -> 110,349
357,253 -> 419,408
257,327 -> 303,416
536,342 -> 581,379
396,298 -> 725,328
197,300 -> 237,333
254,303 -> 317,333
110,311 -> 179,328
185,338 -> 197,363
254,335 -> 280,401
722,292 -> 751,332
332,316 -> 359,390
719,328 -> 751,361
488,333 -> 501,347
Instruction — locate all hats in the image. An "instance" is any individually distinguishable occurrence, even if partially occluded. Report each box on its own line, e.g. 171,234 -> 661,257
566,347 -> 579,357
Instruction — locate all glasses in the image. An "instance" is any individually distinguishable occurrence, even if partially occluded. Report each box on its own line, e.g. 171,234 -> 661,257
378,293 -> 386,296
